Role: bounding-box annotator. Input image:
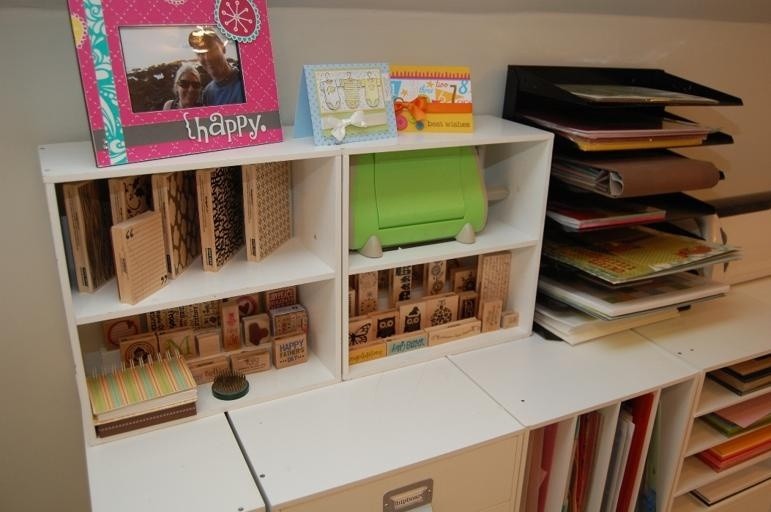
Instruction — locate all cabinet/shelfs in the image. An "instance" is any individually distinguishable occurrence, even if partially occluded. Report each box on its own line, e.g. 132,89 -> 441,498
637,278 -> 769,512
296,97 -> 557,383
444,310 -> 702,508
78,353 -> 269,510
34,117 -> 343,449
496,59 -> 746,345
226,353 -> 527,508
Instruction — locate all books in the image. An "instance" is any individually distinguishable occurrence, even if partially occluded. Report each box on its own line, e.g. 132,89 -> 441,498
526,112 -> 722,150
551,152 -> 720,197
526,357 -> 771,512
55,160 -> 295,305
546,200 -> 666,229
533,292 -> 728,344
536,267 -> 730,321
87,348 -> 198,438
542,225 -> 743,284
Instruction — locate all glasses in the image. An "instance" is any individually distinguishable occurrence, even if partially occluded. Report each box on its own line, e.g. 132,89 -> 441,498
176,79 -> 200,88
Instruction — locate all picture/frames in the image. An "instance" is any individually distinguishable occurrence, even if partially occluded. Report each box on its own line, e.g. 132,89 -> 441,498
60,0 -> 288,169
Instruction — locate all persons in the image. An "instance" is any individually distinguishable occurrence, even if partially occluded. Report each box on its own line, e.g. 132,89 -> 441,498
163,65 -> 203,110
189,29 -> 244,104
